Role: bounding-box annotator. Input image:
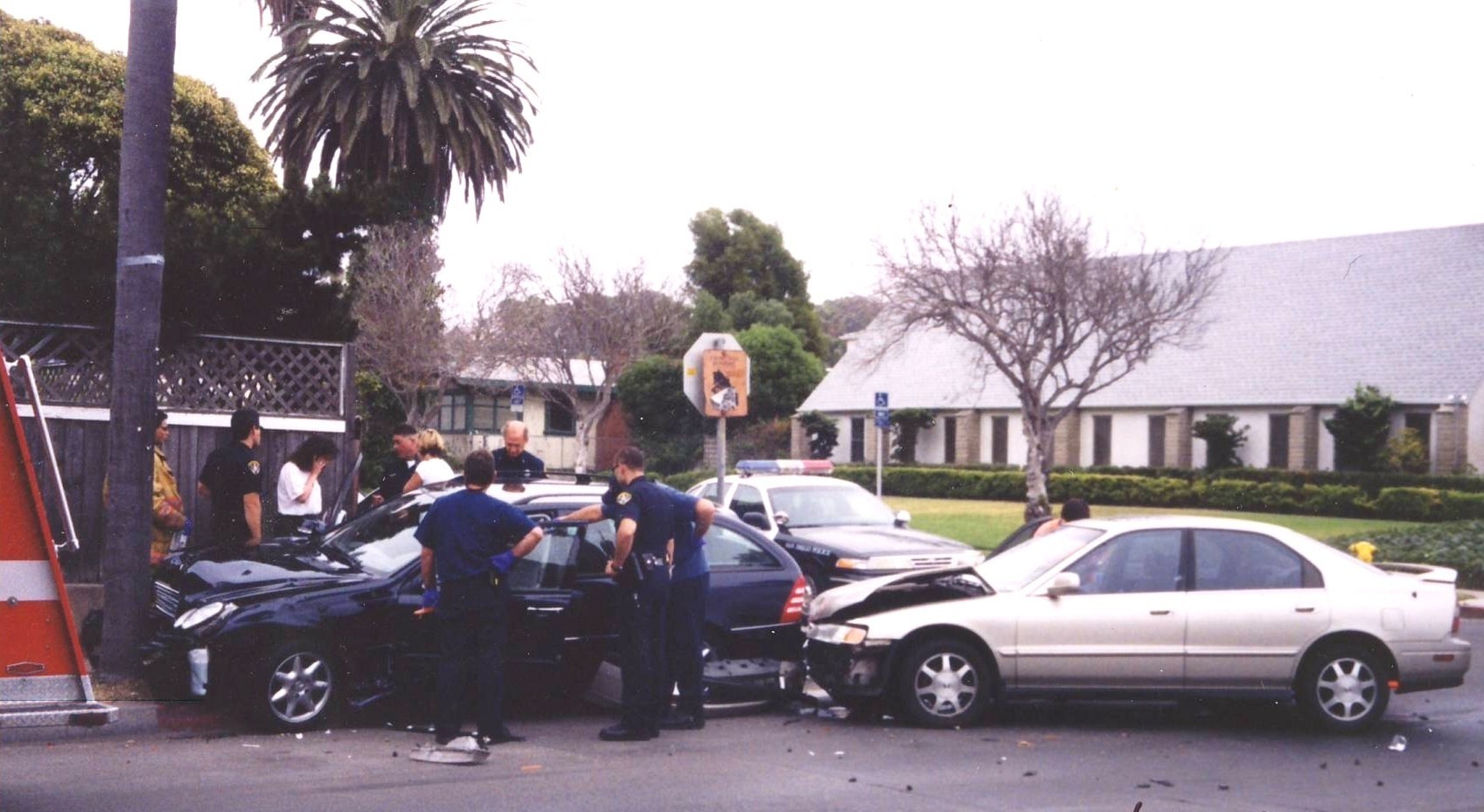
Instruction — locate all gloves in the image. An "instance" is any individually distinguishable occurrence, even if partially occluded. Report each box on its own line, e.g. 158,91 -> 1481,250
420,587 -> 441,608
485,549 -> 518,573
182,517 -> 193,535
546,517 -> 567,537
688,531 -> 705,550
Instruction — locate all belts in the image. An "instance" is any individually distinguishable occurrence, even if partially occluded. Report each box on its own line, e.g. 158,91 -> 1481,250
442,579 -> 500,588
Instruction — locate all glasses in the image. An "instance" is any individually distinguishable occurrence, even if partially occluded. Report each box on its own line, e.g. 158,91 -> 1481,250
158,425 -> 169,432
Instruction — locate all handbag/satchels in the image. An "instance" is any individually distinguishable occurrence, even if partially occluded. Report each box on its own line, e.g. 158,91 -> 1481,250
161,529 -> 187,566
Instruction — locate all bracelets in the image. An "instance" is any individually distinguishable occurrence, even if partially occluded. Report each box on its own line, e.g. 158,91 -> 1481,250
611,561 -> 623,571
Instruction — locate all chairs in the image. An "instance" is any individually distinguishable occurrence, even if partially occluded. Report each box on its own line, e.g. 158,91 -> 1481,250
1219,540 -> 1251,587
1093,542 -> 1131,592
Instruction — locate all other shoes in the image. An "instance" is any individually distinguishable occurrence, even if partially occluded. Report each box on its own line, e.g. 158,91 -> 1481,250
648,721 -> 660,737
479,726 -> 511,744
658,709 -> 705,730
599,713 -> 650,741
435,722 -> 460,743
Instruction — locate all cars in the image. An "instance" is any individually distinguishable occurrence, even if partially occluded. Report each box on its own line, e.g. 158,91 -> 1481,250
143,470 -> 813,733
684,458 -> 984,599
804,513 -> 1472,738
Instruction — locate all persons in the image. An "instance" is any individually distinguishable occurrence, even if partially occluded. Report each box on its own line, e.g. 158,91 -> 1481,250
373,423 -> 454,505
80,409 -> 192,654
275,435 -> 340,538
414,449 -> 544,744
553,447 -> 715,742
1025,493 -> 1091,539
491,420 -> 545,483
197,409 -> 262,548
